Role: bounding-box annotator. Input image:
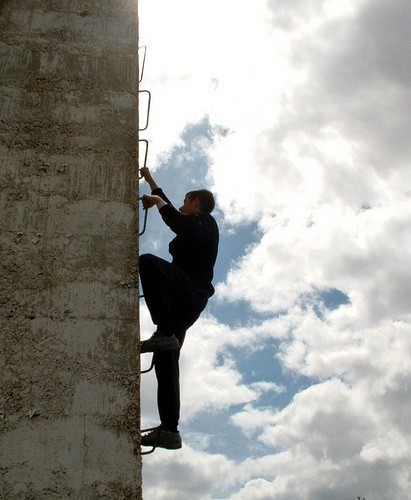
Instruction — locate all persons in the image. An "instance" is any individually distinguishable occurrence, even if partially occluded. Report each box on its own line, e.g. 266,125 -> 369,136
138,168 -> 218,448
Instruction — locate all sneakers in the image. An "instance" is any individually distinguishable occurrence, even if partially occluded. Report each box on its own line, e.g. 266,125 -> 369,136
140,334 -> 179,353
140,424 -> 182,449
